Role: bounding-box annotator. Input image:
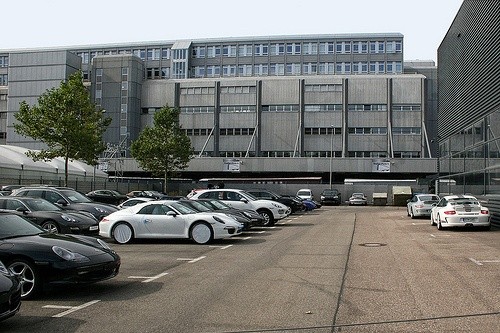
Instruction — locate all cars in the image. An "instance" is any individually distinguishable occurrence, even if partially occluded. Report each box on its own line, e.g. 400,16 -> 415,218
99,200 -> 244,244
0,270 -> 22,322
1,185 -> 25,191
126,190 -> 164,200
430,195 -> 491,230
320,189 -> 341,206
118,197 -> 156,209
197,198 -> 263,229
348,192 -> 368,206
0,197 -> 100,236
0,213 -> 121,300
247,189 -> 303,213
406,194 -> 440,219
160,193 -> 187,201
0,190 -> 11,197
310,200 -> 322,208
300,199 -> 317,213
85,189 -> 134,204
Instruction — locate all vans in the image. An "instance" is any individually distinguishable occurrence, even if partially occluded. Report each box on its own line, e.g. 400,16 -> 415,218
296,188 -> 314,202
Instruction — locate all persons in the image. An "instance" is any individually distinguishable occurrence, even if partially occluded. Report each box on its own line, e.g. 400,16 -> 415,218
191,188 -> 197,196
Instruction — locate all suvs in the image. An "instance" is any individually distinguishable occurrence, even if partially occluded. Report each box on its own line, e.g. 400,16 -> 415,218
9,186 -> 121,221
187,189 -> 291,225
